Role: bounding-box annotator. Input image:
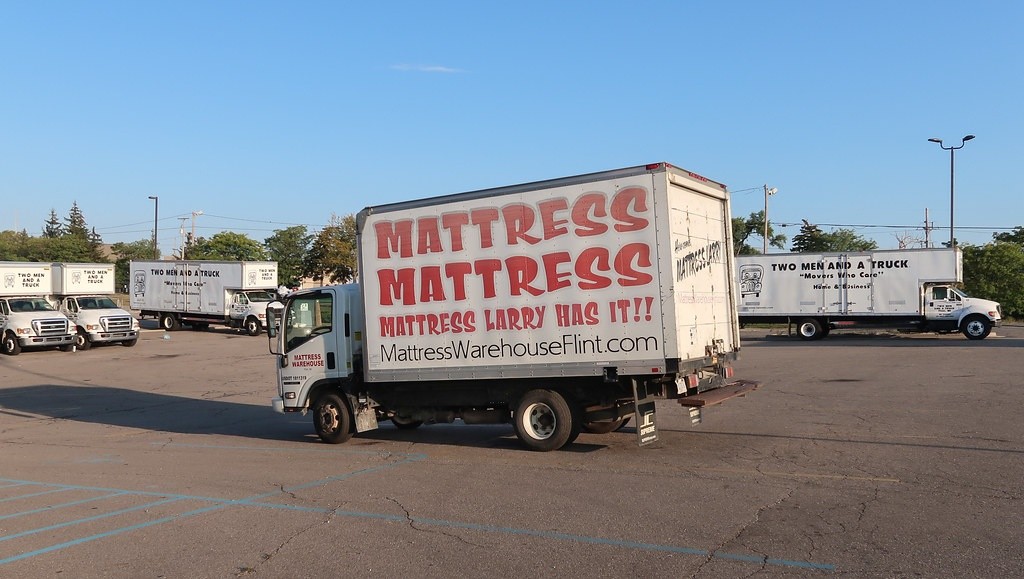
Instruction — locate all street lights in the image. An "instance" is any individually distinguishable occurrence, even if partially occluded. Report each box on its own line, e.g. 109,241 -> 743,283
762,183 -> 780,255
147,196 -> 158,262
928,134 -> 978,249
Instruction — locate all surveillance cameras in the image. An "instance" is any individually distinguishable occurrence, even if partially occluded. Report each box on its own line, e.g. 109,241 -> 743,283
769,191 -> 772,195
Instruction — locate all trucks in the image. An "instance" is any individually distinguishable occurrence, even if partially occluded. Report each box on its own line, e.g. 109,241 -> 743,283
732,247 -> 1004,342
127,258 -> 295,337
37,262 -> 141,351
0,260 -> 78,356
262,156 -> 764,452
